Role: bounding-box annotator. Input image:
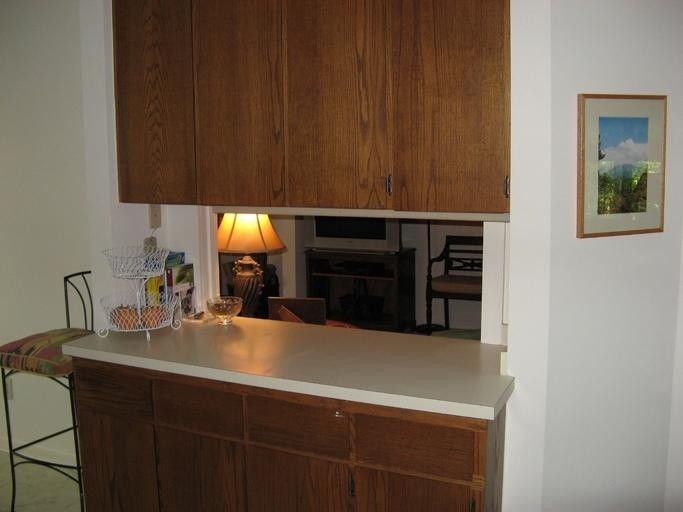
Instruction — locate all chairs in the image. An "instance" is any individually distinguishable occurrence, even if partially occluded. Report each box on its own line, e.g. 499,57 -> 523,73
423,234 -> 484,335
2,270 -> 96,512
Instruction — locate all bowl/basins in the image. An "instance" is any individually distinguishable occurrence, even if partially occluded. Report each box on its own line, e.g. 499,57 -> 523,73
206,296 -> 243,325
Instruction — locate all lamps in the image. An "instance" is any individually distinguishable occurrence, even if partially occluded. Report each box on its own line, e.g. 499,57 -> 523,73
214,212 -> 288,317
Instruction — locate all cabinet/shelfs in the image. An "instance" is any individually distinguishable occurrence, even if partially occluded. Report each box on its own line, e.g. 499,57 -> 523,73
302,248 -> 414,329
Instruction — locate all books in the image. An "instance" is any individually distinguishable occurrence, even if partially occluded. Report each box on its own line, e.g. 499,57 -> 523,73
146,251 -> 195,317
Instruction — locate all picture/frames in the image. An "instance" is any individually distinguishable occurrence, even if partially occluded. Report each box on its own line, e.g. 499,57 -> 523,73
576,93 -> 666,238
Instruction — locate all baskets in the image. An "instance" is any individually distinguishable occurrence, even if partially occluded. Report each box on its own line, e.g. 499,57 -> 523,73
99,245 -> 178,332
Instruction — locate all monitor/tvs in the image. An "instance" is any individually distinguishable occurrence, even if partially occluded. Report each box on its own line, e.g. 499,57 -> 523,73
301,216 -> 402,255
